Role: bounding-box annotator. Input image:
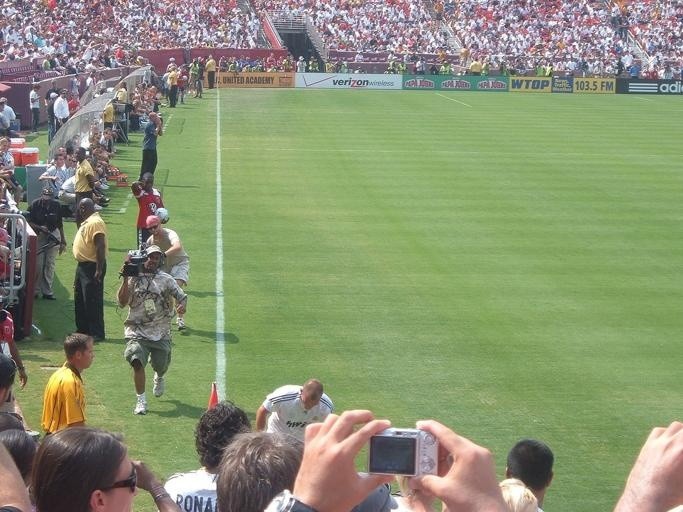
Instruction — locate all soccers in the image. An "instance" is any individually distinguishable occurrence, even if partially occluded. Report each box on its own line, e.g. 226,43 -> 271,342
154,207 -> 169,223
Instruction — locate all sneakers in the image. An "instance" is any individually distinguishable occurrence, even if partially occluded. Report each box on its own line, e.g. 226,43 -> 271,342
177,319 -> 184,329
43,294 -> 56,300
94,183 -> 110,210
134,401 -> 147,414
152,372 -> 164,397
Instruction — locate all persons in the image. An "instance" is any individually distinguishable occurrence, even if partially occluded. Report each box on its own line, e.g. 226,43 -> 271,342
1,0 -> 681,89
1,379 -> 683,511
1,89 -> 204,378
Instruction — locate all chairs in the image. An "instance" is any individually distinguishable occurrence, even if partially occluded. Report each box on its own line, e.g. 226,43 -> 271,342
38,67 -> 158,205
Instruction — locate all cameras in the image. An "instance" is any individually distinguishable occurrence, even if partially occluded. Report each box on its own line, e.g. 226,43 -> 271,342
367,427 -> 439,477
301,58 -> 305,62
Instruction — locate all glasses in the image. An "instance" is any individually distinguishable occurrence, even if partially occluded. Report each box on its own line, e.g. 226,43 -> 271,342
9,359 -> 18,377
145,223 -> 158,230
379,483 -> 390,512
100,463 -> 137,493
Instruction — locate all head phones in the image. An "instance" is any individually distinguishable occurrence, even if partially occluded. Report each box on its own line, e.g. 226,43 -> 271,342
151,249 -> 165,273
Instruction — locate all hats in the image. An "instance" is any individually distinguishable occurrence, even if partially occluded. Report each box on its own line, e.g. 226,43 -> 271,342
145,244 -> 162,255
40,186 -> 54,200
351,472 -> 399,512
146,215 -> 160,225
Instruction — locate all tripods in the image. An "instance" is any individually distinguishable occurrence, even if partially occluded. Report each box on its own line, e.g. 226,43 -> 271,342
113,123 -> 128,144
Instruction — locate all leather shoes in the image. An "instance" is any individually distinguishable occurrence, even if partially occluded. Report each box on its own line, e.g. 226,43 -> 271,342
93,335 -> 103,342
